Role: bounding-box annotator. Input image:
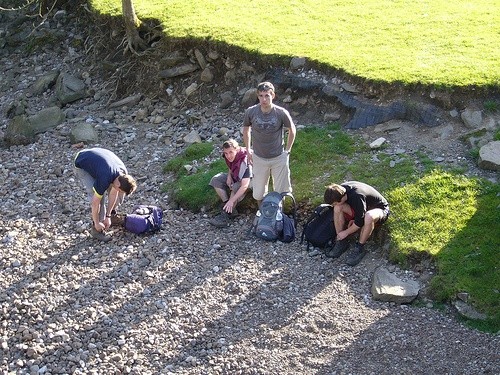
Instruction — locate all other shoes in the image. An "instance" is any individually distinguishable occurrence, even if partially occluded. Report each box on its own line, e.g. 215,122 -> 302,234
90,224 -> 112,242
214,202 -> 238,217
209,214 -> 230,228
111,213 -> 124,225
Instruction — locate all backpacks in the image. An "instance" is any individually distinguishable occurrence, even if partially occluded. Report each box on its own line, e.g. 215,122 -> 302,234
122,204 -> 164,233
252,191 -> 297,242
299,203 -> 335,254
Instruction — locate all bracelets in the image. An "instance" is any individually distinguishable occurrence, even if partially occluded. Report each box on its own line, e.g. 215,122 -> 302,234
285,152 -> 290,154
106,216 -> 111,218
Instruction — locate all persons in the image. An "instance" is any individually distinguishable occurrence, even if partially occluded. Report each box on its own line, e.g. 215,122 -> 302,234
243,82 -> 296,227
74,147 -> 136,241
209,141 -> 253,227
324,181 -> 389,265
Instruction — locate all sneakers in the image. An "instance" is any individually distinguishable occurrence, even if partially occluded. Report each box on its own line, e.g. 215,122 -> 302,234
329,239 -> 349,258
346,243 -> 366,266
